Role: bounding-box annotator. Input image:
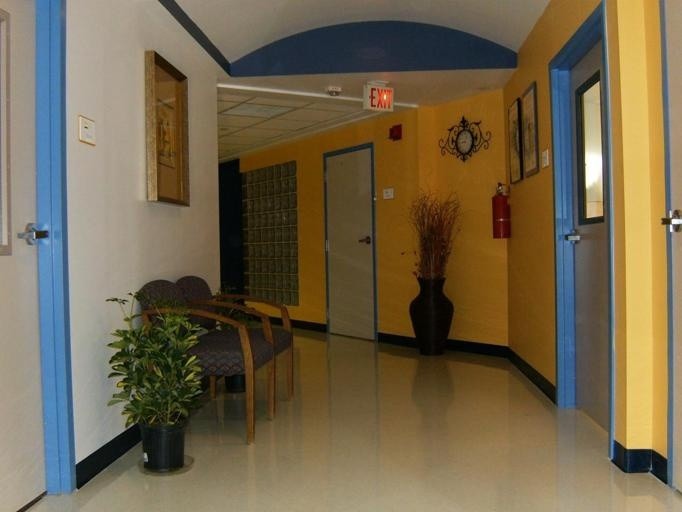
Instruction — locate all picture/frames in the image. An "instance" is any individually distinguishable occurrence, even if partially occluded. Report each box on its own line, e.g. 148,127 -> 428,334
506,97 -> 522,185
144,49 -> 190,207
520,81 -> 542,180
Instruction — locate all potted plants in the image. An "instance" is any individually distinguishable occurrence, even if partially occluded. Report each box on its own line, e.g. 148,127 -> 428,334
105,293 -> 209,473
409,186 -> 462,356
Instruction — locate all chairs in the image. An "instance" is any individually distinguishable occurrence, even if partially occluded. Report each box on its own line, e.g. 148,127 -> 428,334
175,274 -> 296,402
136,280 -> 279,446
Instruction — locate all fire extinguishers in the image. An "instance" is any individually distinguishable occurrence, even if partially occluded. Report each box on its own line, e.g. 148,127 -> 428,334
492,183 -> 511,239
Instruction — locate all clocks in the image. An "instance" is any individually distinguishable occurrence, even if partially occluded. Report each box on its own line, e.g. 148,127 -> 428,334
437,115 -> 492,164
